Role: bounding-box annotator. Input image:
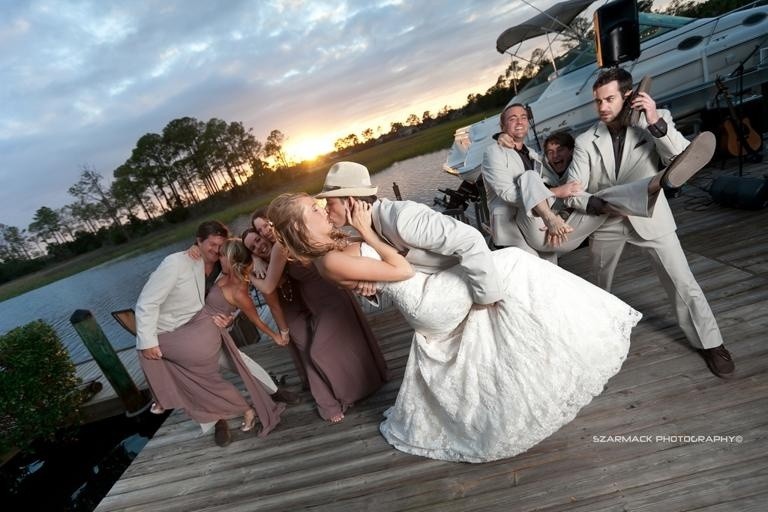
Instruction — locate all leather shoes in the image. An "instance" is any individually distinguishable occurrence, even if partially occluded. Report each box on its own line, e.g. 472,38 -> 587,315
214,419 -> 232,446
272,391 -> 301,407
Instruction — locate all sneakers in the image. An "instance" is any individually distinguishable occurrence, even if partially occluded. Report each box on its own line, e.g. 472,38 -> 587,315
622,75 -> 651,130
663,131 -> 717,189
700,344 -> 735,378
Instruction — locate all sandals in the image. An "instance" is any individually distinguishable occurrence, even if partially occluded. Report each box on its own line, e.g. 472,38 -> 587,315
241,417 -> 256,432
150,403 -> 164,415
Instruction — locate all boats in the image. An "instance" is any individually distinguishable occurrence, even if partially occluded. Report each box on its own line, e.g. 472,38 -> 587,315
442,0 -> 768,187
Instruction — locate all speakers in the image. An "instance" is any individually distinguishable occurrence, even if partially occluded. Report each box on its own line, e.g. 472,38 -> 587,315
593,0 -> 641,68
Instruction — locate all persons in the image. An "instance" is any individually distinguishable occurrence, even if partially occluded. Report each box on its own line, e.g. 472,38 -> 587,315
133,67 -> 737,463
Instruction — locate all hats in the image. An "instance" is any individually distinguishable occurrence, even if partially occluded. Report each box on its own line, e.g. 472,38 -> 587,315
316,158 -> 379,201
